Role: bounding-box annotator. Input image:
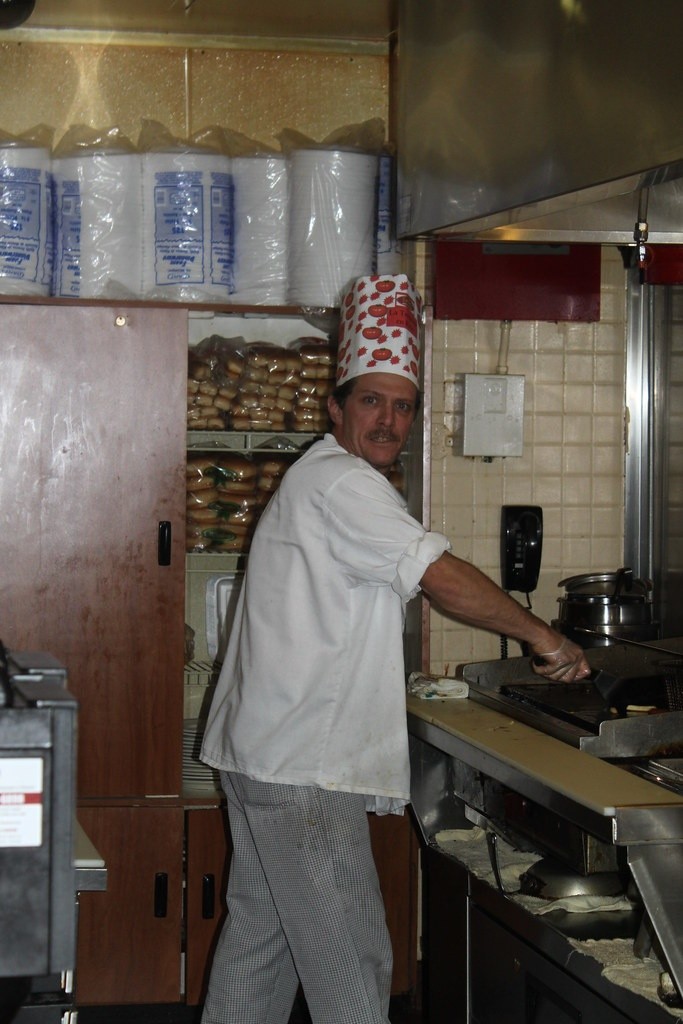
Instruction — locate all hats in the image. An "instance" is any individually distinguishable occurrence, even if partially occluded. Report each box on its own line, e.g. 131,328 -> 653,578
336,273 -> 419,391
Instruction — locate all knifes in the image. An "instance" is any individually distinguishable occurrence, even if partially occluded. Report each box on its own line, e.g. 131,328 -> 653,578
533,654 -> 619,682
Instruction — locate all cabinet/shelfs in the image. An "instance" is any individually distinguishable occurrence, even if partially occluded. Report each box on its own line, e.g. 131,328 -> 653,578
0,293 -> 432,1024
0,650 -> 105,1024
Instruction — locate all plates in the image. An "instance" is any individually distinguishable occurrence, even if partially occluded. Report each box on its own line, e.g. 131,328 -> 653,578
0,148 -> 375,307
182,718 -> 221,790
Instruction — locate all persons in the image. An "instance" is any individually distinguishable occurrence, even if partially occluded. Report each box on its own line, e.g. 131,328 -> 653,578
199,273 -> 591,1024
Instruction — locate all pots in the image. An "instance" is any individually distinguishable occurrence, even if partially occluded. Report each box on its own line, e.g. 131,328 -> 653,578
551,568 -> 660,649
488,833 -> 626,901
